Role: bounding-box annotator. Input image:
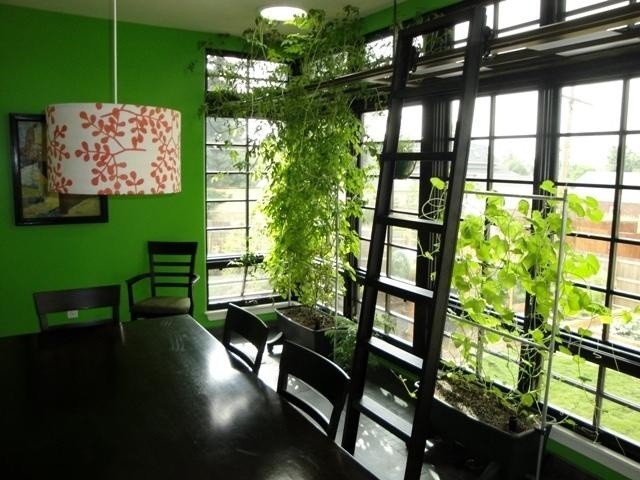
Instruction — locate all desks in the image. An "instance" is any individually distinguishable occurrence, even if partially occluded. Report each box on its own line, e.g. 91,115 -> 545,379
1,315 -> 385,479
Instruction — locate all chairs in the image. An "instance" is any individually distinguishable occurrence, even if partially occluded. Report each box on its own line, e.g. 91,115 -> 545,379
222,302 -> 269,376
276,339 -> 351,442
126,241 -> 201,321
32,283 -> 126,331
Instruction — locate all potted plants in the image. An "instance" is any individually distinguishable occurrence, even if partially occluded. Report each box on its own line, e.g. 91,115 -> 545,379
187,4 -> 454,355
392,175 -> 640,480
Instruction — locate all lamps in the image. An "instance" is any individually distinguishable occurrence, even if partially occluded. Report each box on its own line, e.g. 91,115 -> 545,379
46,0 -> 181,196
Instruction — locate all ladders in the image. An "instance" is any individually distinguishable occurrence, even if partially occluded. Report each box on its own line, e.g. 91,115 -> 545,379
341,10 -> 485,480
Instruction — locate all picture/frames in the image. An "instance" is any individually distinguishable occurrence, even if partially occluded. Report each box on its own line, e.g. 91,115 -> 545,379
9,112 -> 110,227
